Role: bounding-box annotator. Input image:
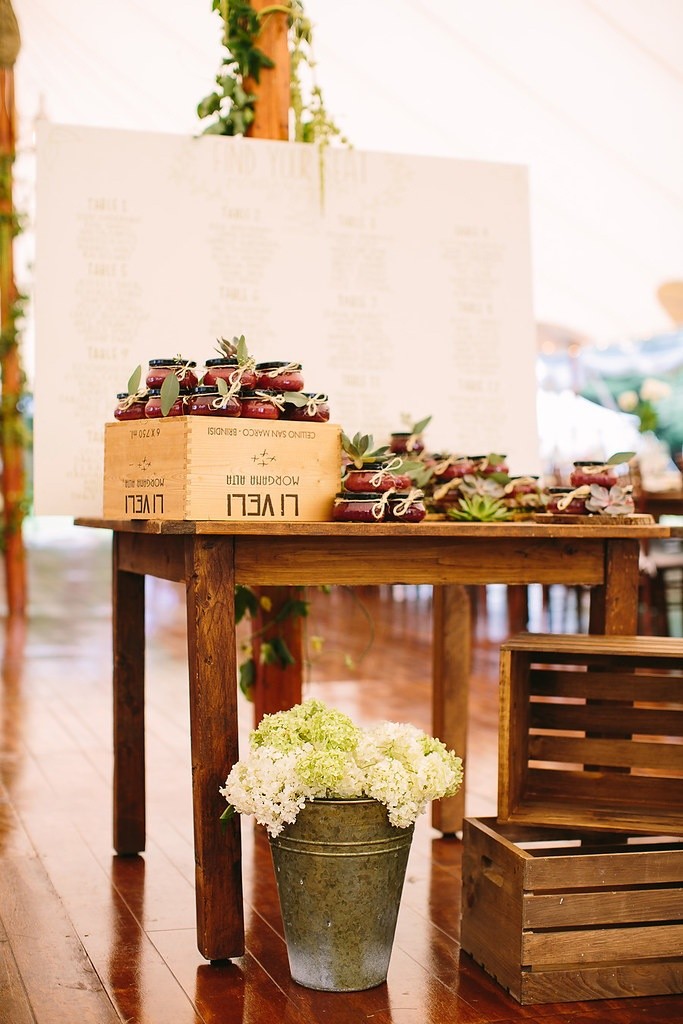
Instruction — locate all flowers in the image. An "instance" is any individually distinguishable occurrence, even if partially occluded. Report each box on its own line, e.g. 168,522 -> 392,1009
218,702 -> 465,838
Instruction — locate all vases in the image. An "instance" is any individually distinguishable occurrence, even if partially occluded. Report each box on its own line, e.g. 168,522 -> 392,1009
267,800 -> 415,990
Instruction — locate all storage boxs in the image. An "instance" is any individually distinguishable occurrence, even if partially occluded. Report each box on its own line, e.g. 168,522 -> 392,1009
104,415 -> 344,524
461,816 -> 683,1004
497,632 -> 683,838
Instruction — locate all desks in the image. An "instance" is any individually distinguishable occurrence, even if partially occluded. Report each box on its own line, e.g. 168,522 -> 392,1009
73,516 -> 669,962
506,486 -> 683,635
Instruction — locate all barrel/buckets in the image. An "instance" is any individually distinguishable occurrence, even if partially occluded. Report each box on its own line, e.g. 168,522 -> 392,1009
269,799 -> 415,991
